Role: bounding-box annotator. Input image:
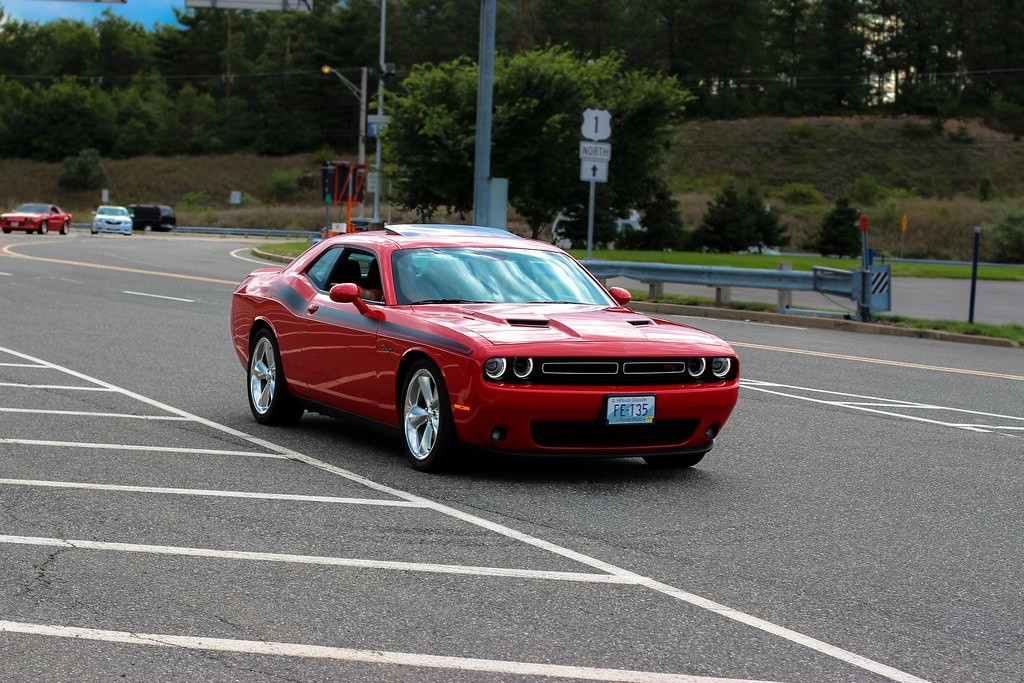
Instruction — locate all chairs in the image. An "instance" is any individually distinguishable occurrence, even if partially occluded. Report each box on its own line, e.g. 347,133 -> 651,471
339,256 -> 382,290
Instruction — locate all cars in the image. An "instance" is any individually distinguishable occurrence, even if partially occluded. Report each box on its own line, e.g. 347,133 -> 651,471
92,206 -> 136,235
0,202 -> 73,235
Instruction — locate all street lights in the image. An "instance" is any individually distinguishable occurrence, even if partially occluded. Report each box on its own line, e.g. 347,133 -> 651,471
321,64 -> 368,220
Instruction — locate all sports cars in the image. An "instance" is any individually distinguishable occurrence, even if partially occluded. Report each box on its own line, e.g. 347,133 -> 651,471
230,222 -> 742,478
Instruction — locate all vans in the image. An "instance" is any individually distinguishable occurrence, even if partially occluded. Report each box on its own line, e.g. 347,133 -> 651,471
127,204 -> 178,230
